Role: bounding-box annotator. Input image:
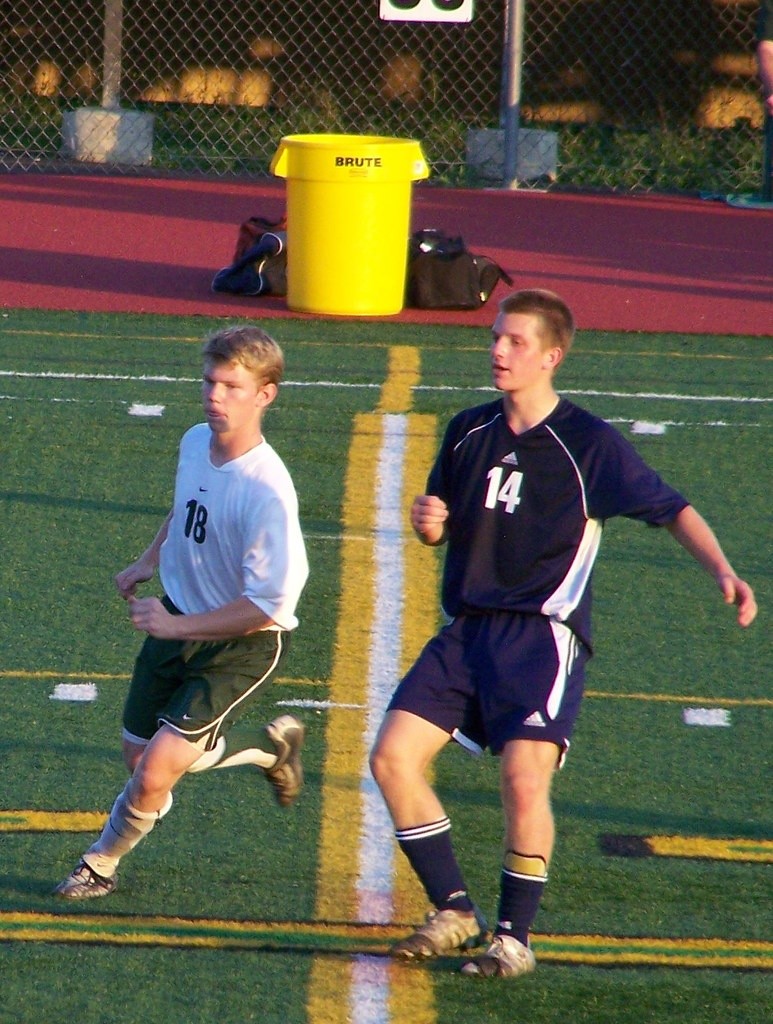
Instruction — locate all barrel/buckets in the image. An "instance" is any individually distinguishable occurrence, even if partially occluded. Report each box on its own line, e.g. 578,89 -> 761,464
269,133 -> 429,317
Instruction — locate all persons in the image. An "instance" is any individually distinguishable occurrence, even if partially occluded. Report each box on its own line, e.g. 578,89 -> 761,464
757,0 -> 772,115
49,327 -> 309,902
368,289 -> 759,979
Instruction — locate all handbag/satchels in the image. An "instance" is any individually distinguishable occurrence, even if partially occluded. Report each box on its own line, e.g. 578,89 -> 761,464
407,227 -> 514,309
211,214 -> 287,297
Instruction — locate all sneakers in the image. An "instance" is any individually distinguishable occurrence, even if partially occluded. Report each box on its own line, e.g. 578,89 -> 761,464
263,715 -> 304,806
52,856 -> 120,900
389,904 -> 488,959
461,934 -> 535,979
